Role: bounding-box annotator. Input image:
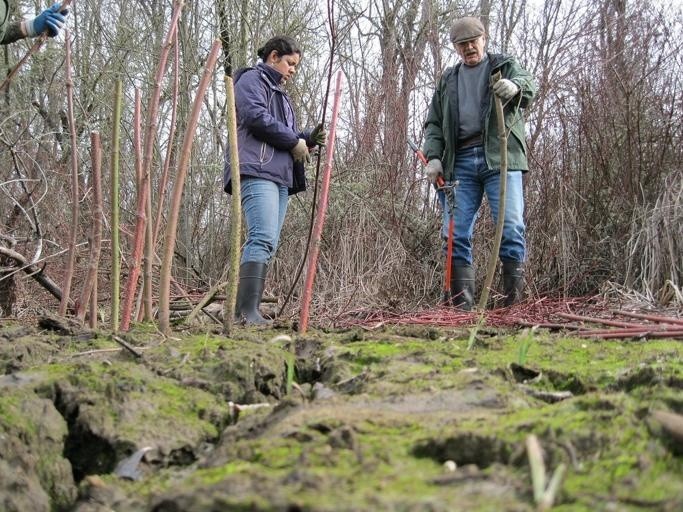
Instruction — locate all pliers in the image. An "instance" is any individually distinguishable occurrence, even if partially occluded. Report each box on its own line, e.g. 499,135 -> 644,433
404,138 -> 460,306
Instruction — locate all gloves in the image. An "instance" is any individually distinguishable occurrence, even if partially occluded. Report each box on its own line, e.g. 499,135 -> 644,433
493,78 -> 520,99
24,1 -> 69,37
425,159 -> 443,185
291,123 -> 326,164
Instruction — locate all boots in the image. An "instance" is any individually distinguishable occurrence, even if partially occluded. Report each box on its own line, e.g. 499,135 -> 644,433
450,265 -> 475,311
235,262 -> 271,325
502,262 -> 524,303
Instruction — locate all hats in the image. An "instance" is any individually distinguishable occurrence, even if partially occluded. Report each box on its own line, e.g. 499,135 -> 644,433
449,16 -> 485,44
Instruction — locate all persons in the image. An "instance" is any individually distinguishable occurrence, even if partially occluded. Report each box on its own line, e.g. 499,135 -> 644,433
222,36 -> 327,325
422,16 -> 535,309
0,0 -> 70,46
